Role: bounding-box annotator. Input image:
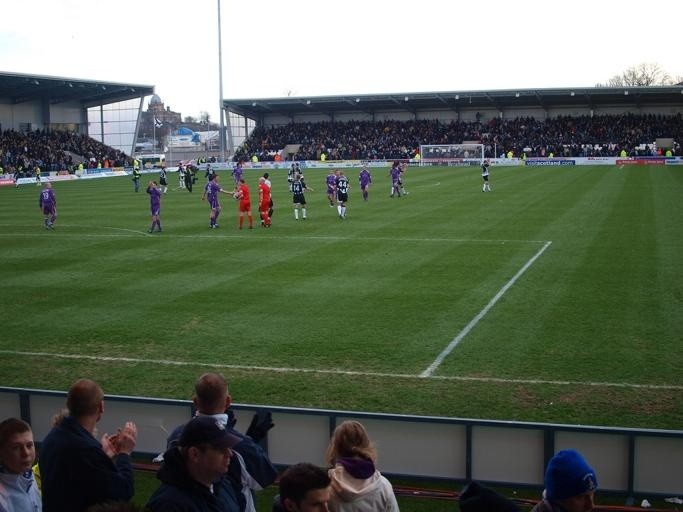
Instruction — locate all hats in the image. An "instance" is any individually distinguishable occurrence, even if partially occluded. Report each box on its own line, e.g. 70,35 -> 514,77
179,416 -> 243,448
545,449 -> 597,499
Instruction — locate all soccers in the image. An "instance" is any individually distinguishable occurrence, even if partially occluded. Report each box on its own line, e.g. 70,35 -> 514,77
234,193 -> 240,198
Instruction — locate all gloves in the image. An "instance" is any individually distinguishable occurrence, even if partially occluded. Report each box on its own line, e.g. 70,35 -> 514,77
245,409 -> 275,443
223,410 -> 237,429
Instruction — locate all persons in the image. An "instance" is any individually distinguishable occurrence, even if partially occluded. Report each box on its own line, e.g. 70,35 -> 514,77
201,163 -> 273,229
0,127 -> 141,193
39,183 -> 57,231
144,415 -> 244,512
274,462 -> 332,512
235,112 -> 682,162
152,373 -> 278,512
161,154 -> 221,194
326,420 -> 400,512
32,408 -> 97,498
88,500 -> 152,512
146,181 -> 161,233
0,417 -> 42,512
289,162 -> 313,221
326,161 -> 408,220
38,379 -> 138,512
531,448 -> 598,512
481,160 -> 491,192
459,482 -> 520,512
